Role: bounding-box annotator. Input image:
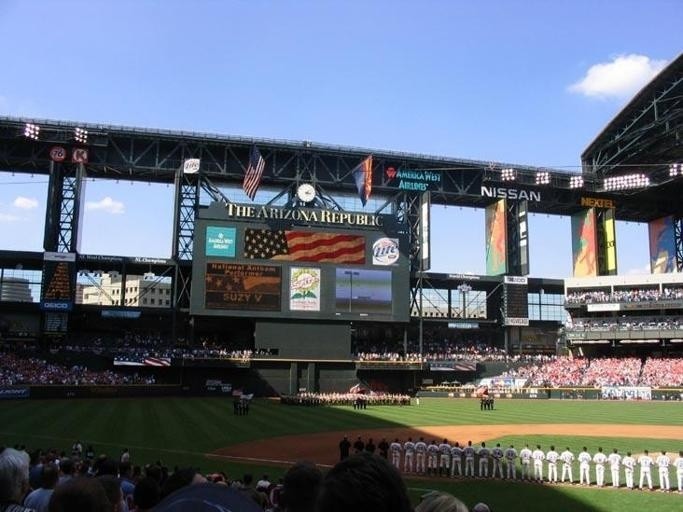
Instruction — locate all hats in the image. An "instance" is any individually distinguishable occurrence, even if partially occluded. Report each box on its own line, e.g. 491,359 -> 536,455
150,483 -> 260,512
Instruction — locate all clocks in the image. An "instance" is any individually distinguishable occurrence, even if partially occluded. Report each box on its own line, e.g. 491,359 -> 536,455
296,182 -> 316,203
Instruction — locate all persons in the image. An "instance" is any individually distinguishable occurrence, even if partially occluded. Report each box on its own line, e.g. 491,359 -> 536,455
479,397 -> 494,410
279,390 -> 349,410
353,397 -> 367,410
232,389 -> 255,416
0,313 -> 274,389
0,434 -> 493,512
493,442 -> 683,493
349,288 -> 681,400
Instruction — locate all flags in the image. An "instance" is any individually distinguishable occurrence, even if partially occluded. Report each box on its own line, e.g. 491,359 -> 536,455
350,154 -> 372,207
239,142 -> 266,202
